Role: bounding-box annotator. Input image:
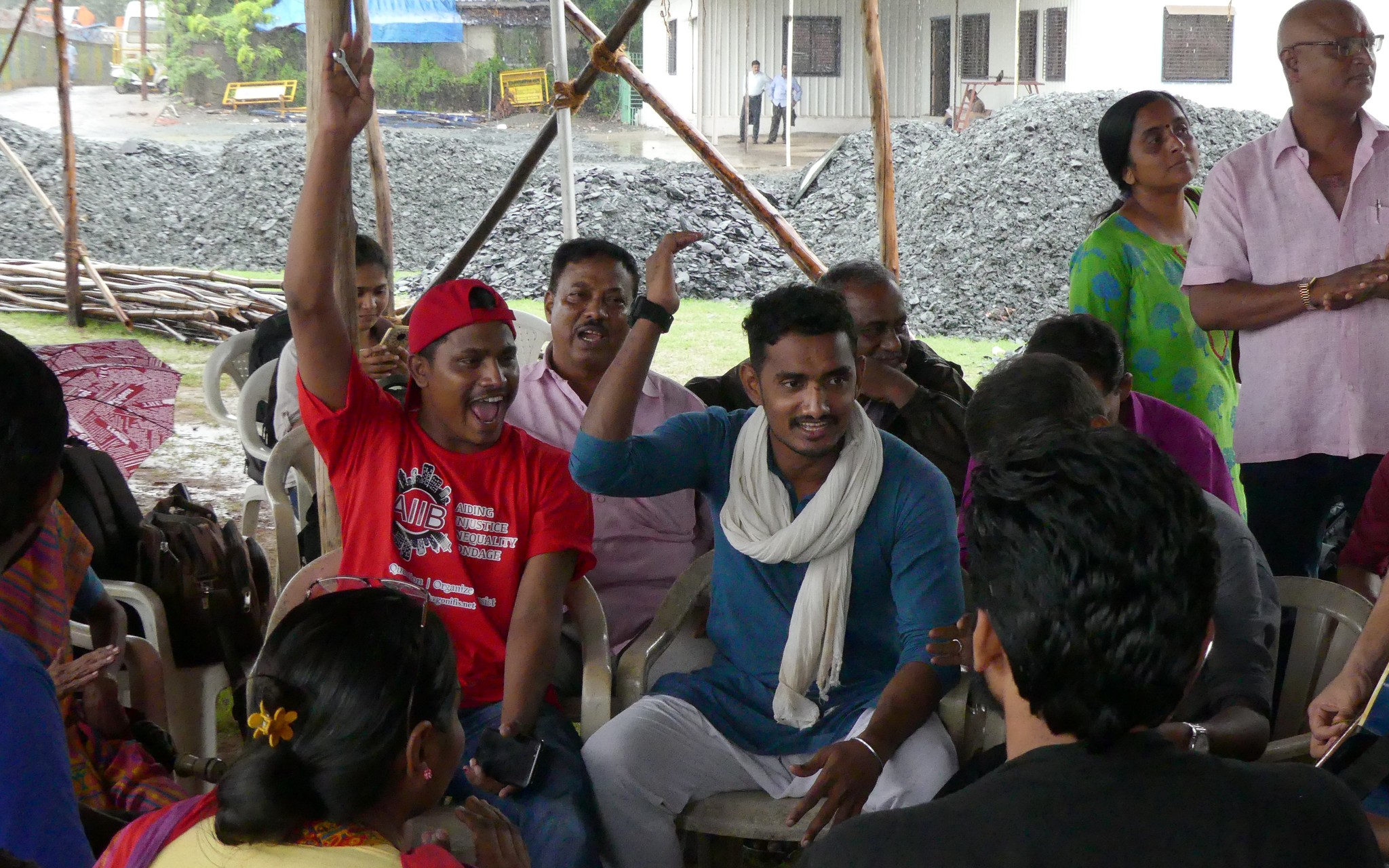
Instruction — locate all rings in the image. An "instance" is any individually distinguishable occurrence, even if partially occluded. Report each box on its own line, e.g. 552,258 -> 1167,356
950,638 -> 963,652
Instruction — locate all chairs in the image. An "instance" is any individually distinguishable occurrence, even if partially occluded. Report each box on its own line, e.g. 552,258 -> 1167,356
68,311 -> 1373,844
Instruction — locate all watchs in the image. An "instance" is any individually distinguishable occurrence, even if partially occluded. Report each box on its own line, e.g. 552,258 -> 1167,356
627,295 -> 674,333
1297,276 -> 1318,310
1182,721 -> 1210,755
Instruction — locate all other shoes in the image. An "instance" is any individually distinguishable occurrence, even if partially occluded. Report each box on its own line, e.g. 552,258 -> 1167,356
763,140 -> 774,145
782,139 -> 786,145
736,138 -> 745,144
752,140 -> 757,144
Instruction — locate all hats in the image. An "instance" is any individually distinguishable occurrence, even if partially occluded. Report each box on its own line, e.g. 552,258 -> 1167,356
407,277 -> 517,355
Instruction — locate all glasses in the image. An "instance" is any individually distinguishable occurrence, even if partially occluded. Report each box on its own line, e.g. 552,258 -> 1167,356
304,574 -> 431,741
1292,34 -> 1384,58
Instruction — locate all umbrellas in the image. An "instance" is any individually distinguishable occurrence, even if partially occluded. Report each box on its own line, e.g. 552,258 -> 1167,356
28,339 -> 186,481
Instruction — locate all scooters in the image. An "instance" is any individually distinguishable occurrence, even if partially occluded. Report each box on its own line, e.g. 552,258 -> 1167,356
109,48 -> 177,94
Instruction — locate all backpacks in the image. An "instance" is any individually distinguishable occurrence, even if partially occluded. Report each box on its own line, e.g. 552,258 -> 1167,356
139,480 -> 273,668
55,434 -> 150,582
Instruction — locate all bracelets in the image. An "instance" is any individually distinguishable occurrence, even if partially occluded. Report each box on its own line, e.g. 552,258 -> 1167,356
850,737 -> 883,772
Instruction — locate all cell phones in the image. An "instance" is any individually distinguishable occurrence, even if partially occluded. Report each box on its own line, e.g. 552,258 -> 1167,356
475,728 -> 544,789
371,325 -> 409,373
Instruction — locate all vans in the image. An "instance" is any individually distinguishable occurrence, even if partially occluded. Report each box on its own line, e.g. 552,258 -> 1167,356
121,0 -> 168,62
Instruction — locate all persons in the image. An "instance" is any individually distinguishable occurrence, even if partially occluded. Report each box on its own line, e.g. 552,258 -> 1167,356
1,496 -> 194,819
1066,88 -> 1247,525
795,422 -> 1385,868
503,239 -> 711,720
283,28 -> 600,868
570,233 -> 963,868
249,234 -> 411,524
958,310 -> 1240,576
765,63 -> 802,145
1181,0 -> 1388,867
737,60 -> 773,145
681,258 -> 976,507
0,330 -> 91,868
89,572 -> 531,868
926,353 -> 1282,761
